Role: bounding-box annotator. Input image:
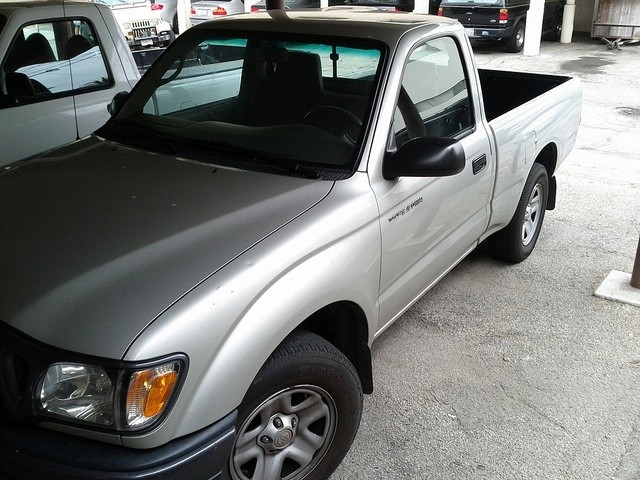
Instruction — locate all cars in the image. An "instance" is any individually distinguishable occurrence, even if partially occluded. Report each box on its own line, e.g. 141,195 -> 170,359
326,0 -> 414,12
251,0 -> 320,13
188,0 -> 244,29
104,0 -> 179,53
437,0 -> 567,53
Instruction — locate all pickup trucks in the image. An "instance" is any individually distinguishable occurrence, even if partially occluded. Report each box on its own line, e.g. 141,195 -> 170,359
0,1 -> 247,169
0,5 -> 584,480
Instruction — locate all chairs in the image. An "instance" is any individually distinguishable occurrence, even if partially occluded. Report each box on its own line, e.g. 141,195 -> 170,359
262,50 -> 326,120
65,35 -> 93,60
20,32 -> 56,68
402,59 -> 459,138
